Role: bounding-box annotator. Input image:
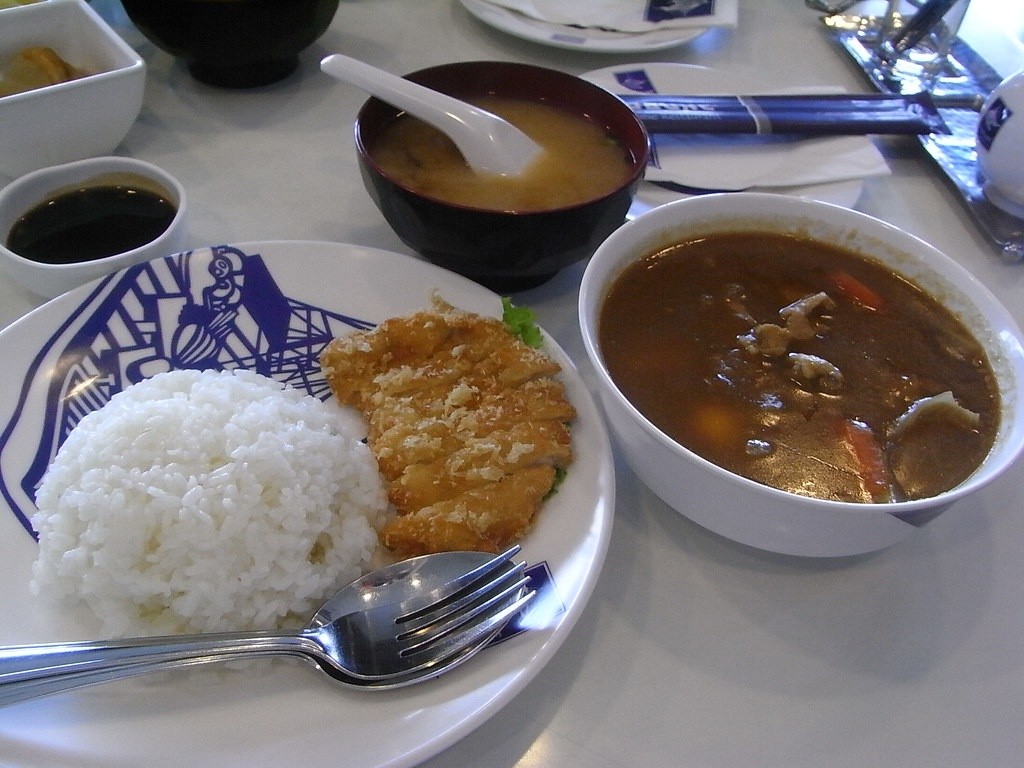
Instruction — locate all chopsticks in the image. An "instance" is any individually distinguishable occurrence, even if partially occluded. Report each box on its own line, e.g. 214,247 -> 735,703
614,91 -> 954,135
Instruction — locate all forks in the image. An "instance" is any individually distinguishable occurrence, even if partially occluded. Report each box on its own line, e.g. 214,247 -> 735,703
0,544 -> 537,680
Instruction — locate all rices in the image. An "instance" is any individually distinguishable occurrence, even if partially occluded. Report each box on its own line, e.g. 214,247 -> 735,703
28,366 -> 396,673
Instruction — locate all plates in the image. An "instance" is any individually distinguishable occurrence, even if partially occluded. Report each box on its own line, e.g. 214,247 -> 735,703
579,62 -> 868,209
821,13 -> 1024,256
461,0 -> 714,52
0,240 -> 616,768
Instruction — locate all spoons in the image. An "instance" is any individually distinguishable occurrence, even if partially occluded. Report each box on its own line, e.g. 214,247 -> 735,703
319,54 -> 546,176
0,551 -> 526,710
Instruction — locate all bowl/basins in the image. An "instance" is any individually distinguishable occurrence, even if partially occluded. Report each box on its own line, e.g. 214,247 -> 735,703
120,0 -> 340,89
579,192 -> 1023,557
353,60 -> 651,296
0,156 -> 187,299
0,0 -> 146,178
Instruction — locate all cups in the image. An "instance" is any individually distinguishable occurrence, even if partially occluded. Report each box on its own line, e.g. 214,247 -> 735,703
874,0 -> 972,78
973,66 -> 1024,221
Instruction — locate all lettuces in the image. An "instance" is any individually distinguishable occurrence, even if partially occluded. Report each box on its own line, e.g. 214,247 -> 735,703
498,295 -> 567,502
